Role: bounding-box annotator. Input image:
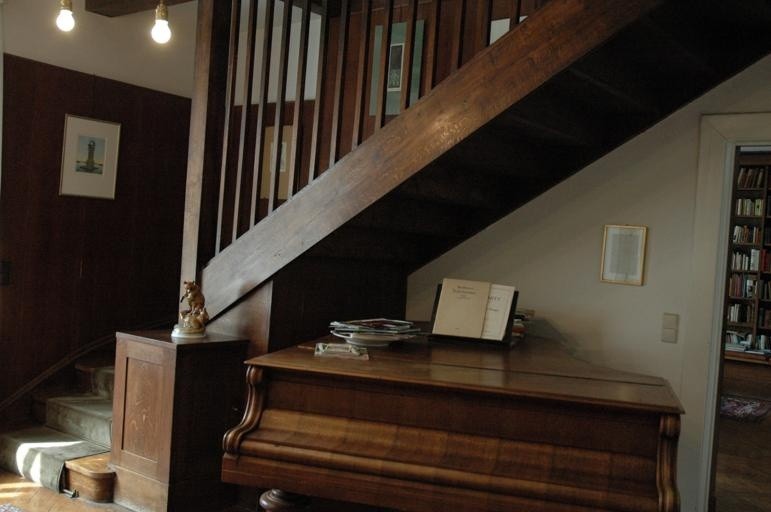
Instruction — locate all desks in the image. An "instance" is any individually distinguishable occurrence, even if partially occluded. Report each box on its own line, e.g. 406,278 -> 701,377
219,336 -> 686,512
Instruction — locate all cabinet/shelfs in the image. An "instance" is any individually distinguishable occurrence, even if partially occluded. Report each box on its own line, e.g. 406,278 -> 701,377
721,151 -> 771,401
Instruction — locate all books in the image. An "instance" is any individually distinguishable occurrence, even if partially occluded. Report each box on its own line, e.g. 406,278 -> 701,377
725,168 -> 770,360
430,278 -> 534,346
326,315 -> 421,347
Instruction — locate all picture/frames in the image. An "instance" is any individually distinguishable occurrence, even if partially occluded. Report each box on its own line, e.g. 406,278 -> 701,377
600,224 -> 647,286
58,113 -> 122,199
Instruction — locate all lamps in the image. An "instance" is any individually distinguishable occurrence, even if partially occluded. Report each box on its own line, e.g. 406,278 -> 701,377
151,0 -> 172,45
56,0 -> 76,32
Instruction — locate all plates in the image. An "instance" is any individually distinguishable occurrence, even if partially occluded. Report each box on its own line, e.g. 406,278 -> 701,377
331,330 -> 417,347
